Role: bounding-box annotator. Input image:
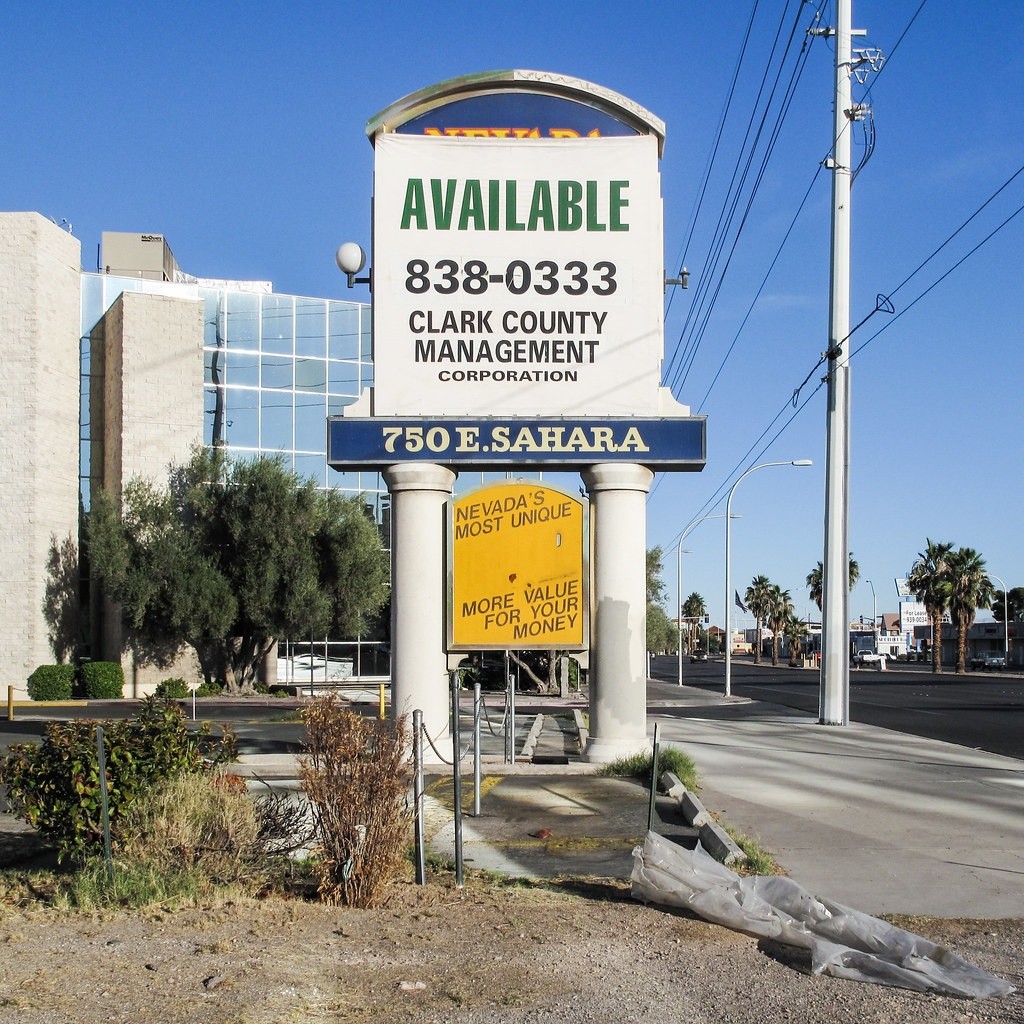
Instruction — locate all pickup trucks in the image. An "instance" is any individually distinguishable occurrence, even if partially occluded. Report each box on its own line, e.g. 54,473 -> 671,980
853,649 -> 878,666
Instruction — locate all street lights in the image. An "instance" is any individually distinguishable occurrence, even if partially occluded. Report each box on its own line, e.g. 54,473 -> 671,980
866,580 -> 876,629
723,459 -> 812,697
979,573 -> 1009,666
677,514 -> 743,688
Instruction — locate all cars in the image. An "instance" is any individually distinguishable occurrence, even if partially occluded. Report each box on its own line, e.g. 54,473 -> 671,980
691,650 -> 708,664
971,651 -> 1006,671
